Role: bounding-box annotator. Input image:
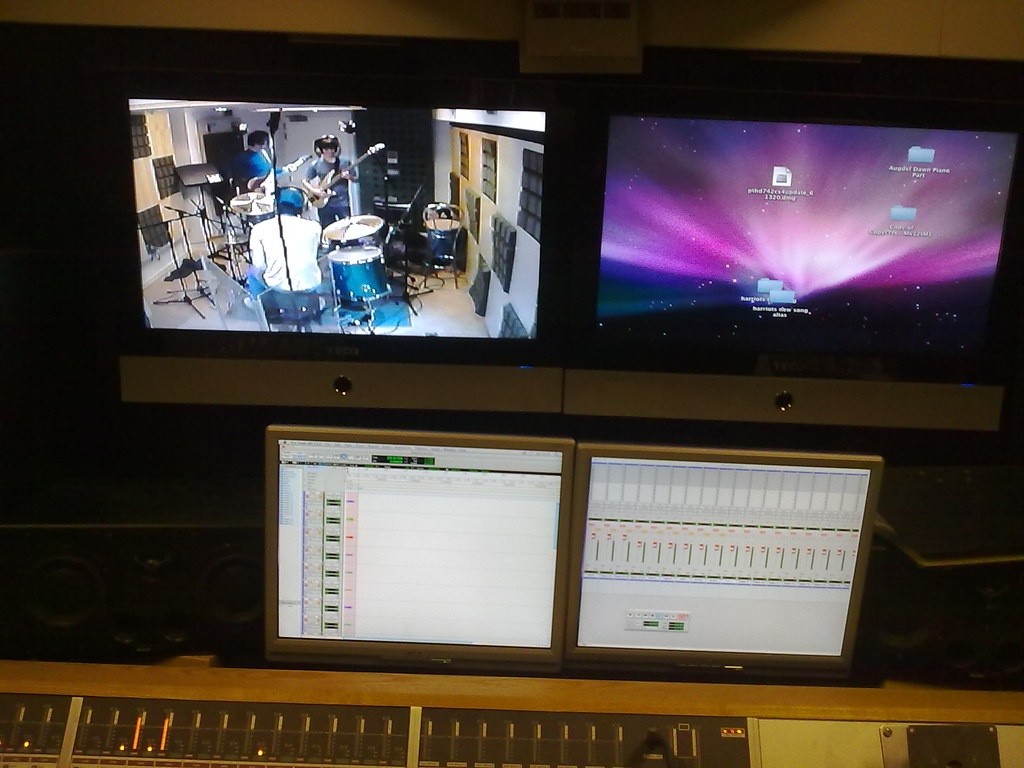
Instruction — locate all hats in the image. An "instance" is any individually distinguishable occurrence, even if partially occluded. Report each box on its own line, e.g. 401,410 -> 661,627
275,188 -> 304,207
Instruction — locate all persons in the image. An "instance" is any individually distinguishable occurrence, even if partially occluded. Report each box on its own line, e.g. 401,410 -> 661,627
303,135 -> 359,229
242,187 -> 321,312
224,130 -> 298,193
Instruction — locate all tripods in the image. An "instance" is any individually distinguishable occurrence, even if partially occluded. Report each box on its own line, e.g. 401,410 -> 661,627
138,206 -> 247,315
394,184 -> 434,315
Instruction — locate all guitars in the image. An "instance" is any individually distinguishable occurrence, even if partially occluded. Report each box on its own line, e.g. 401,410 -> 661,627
247,154 -> 311,192
305,142 -> 387,208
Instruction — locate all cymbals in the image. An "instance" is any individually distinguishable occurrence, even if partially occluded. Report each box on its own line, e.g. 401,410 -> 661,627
230,192 -> 275,216
322,214 -> 385,242
208,234 -> 248,245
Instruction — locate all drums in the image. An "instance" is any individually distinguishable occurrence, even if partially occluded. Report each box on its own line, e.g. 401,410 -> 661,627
327,244 -> 392,303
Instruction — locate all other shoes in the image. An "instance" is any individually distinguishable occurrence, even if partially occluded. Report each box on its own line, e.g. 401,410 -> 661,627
244,297 -> 256,312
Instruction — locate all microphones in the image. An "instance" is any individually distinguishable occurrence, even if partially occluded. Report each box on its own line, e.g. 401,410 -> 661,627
338,121 -> 354,134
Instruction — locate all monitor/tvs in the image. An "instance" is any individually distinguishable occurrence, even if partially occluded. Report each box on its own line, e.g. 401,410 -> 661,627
90,62 -> 1024,687
